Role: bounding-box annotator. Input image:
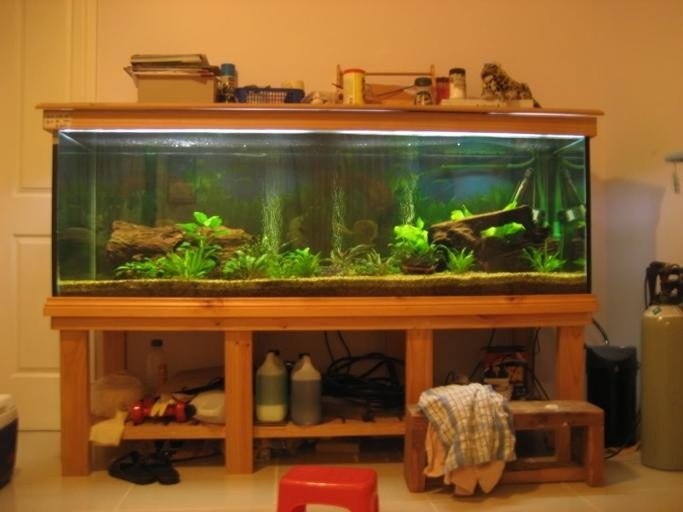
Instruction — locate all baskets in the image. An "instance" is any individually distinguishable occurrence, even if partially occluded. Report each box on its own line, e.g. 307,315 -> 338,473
235,87 -> 304,104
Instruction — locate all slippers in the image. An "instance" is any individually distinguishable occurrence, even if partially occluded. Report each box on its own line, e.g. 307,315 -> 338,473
108,450 -> 155,485
139,454 -> 180,484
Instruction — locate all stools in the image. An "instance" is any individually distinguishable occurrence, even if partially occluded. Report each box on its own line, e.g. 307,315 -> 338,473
275,464 -> 379,511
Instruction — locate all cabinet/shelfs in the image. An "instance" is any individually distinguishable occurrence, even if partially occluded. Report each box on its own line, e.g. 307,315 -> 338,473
41,295 -> 601,477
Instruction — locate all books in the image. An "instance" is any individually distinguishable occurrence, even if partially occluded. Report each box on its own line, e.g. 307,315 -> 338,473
437,94 -> 536,110
121,51 -> 222,84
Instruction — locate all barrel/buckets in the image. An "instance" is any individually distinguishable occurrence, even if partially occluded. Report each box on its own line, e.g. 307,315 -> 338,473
255,350 -> 288,422
290,351 -> 323,424
185,390 -> 224,421
143,338 -> 174,392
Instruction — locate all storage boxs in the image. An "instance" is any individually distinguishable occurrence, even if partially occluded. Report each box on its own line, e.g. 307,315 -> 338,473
122,52 -> 220,104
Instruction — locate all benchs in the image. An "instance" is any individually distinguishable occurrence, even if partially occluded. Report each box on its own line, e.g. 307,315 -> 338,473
401,399 -> 606,492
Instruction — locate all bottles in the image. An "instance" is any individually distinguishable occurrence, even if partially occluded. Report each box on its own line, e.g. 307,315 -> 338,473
415,66 -> 467,106
144,339 -> 168,399
341,66 -> 366,103
220,62 -> 236,103
